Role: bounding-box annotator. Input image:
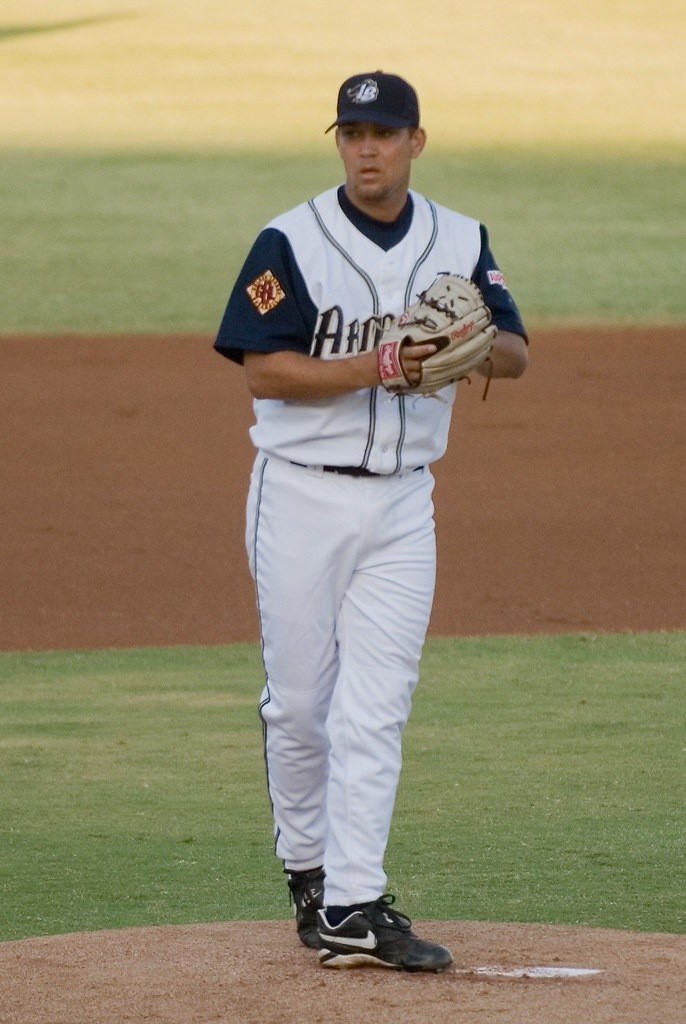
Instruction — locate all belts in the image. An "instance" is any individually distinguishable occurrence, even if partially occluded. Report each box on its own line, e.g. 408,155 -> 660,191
290,461 -> 423,477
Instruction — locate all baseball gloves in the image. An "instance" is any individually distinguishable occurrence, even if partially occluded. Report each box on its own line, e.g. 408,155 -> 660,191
375,271 -> 503,396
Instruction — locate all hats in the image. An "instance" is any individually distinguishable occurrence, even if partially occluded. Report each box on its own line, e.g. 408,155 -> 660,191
325,72 -> 419,129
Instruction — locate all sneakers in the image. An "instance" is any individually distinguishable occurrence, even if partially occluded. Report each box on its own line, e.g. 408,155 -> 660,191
318,894 -> 454,972
287,867 -> 326,949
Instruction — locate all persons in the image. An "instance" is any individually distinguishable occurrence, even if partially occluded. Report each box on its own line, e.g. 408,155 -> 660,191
211,70 -> 528,972
